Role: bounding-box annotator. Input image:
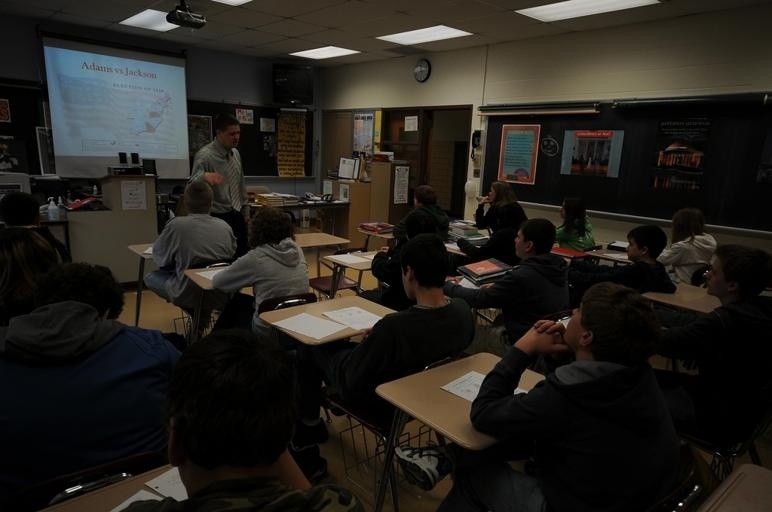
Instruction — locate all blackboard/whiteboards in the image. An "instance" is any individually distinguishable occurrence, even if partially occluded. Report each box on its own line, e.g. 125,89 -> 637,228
477,91 -> 772,238
186,97 -> 318,181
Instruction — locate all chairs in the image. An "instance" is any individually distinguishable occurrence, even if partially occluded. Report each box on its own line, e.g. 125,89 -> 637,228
172,263 -> 230,344
323,356 -> 447,512
307,246 -> 368,302
677,416 -> 771,468
652,446 -> 734,512
257,289 -> 318,314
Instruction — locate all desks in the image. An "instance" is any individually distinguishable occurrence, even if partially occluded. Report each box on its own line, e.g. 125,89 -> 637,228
321,249 -> 390,298
294,232 -> 351,277
242,191 -> 352,250
694,463 -> 772,512
180,263 -> 253,345
127,243 -> 153,327
257,294 -> 400,422
56,438 -> 313,512
585,246 -> 634,265
356,220 -> 397,288
638,281 -> 723,314
452,275 -> 495,330
368,351 -> 547,511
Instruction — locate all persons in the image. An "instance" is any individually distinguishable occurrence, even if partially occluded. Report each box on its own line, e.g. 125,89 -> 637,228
443,218 -> 570,345
554,194 -> 595,251
189,114 -> 251,257
0,262 -> 181,512
113,351 -> 365,512
0,226 -> 58,323
652,244 -> 768,449
475,181 -> 529,230
288,232 -> 476,443
395,282 -> 681,511
0,192 -> 69,262
657,208 -> 715,284
572,225 -> 677,306
184,206 -> 308,346
391,185 -> 452,239
143,181 -> 236,304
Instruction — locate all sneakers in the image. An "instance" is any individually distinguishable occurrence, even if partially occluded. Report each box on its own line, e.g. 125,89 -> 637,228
394,444 -> 447,491
304,417 -> 329,445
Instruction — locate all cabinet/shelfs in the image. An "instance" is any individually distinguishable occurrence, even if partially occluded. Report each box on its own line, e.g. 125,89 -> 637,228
323,177 -> 372,251
29,174 -> 160,290
360,157 -> 392,252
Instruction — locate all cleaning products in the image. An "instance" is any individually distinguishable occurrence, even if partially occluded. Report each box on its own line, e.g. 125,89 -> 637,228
48,196 -> 59,222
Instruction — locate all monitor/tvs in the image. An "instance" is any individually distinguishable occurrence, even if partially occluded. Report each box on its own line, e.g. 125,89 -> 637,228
270,62 -> 315,106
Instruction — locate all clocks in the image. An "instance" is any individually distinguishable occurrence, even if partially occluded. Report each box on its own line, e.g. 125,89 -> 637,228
413,57 -> 432,83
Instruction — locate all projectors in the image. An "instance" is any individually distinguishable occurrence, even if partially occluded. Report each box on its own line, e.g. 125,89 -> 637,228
167,4 -> 208,30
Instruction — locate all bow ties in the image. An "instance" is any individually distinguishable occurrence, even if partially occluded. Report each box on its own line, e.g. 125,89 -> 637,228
226,154 -> 242,212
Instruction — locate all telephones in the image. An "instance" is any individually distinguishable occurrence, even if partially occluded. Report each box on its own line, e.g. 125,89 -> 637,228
471,130 -> 481,158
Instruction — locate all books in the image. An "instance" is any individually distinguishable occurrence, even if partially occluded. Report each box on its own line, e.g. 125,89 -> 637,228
255,193 -> 298,205
